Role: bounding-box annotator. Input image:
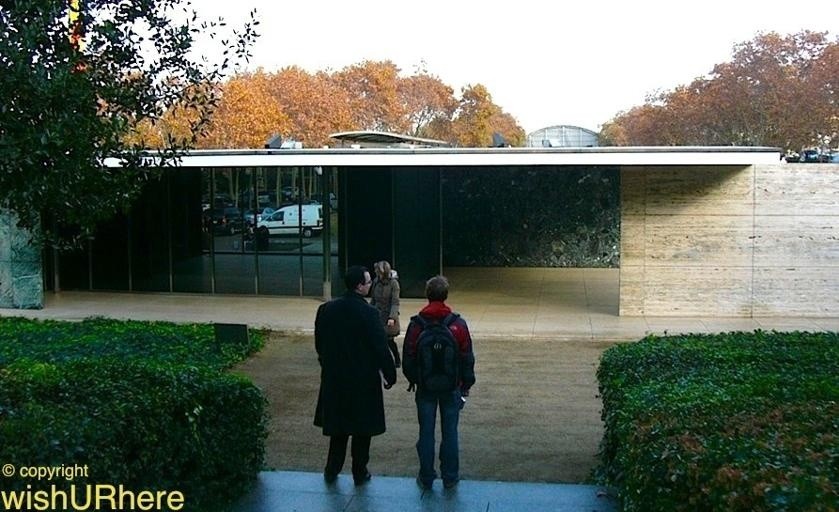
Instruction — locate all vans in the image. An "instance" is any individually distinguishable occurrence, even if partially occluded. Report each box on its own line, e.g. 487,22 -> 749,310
253,203 -> 325,240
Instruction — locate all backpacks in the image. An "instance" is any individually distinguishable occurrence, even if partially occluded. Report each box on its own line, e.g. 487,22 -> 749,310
411,312 -> 460,393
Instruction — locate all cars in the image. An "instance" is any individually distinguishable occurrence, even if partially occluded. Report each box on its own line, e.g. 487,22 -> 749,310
199,187 -> 338,226
786,148 -> 832,163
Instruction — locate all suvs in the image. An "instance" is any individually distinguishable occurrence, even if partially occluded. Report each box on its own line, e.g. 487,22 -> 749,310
202,207 -> 245,236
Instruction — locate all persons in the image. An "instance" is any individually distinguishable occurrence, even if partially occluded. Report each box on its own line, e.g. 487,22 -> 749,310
369,261 -> 399,368
402,275 -> 476,488
314,265 -> 396,485
